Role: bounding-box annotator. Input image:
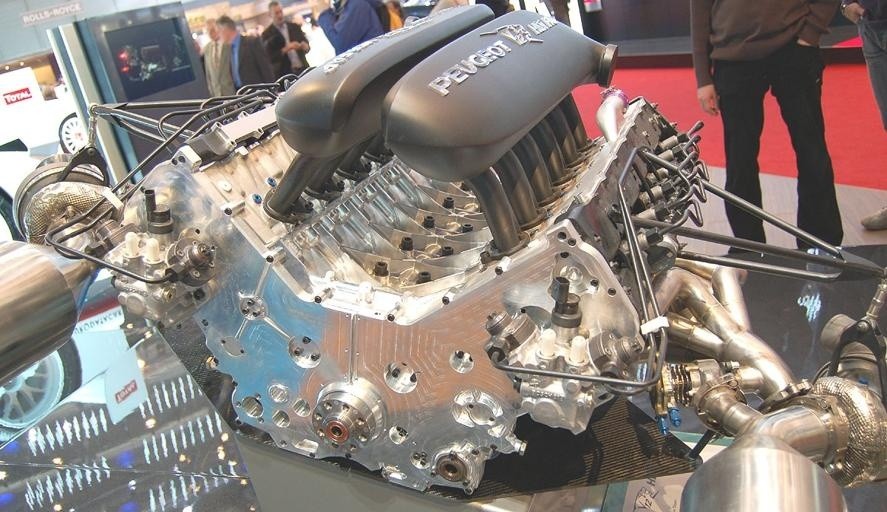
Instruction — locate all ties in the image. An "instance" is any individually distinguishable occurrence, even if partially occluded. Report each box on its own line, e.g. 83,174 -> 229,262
213,41 -> 220,65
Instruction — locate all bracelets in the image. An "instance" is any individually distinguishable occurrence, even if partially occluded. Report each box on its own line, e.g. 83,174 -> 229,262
838,0 -> 856,16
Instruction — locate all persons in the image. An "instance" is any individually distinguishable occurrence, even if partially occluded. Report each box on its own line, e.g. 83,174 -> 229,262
188,0 -> 405,104
839,0 -> 886,232
687,1 -> 844,254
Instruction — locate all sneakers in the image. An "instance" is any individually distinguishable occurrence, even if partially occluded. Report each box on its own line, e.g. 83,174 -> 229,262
859,207 -> 886,230
